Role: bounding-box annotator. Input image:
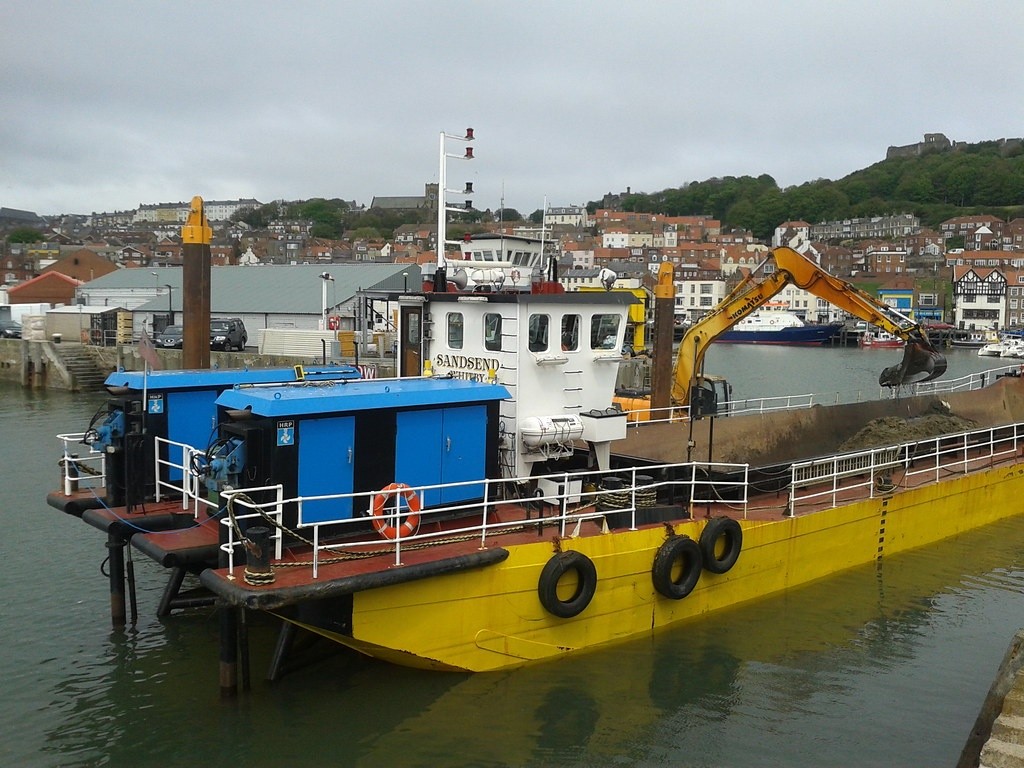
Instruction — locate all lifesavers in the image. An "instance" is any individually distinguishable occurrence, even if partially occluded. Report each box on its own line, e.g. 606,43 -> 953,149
537,550 -> 597,618
652,534 -> 703,600
371,483 -> 420,538
699,516 -> 743,574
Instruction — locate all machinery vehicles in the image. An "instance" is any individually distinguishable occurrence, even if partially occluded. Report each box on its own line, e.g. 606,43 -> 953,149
611,243 -> 950,427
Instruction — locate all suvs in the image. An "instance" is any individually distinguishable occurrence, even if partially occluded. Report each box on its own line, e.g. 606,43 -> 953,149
209,317 -> 248,352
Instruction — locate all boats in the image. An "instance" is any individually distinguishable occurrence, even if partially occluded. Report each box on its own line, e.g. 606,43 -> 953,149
977,338 -> 1024,359
709,294 -> 849,347
856,326 -> 908,348
42,127 -> 1024,679
238,246 -> 260,267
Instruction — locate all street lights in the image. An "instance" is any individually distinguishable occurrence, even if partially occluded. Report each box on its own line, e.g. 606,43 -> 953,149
152,272 -> 158,297
164,284 -> 171,312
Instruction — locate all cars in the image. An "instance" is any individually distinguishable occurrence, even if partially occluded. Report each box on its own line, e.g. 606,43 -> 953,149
154,325 -> 183,349
925,322 -> 955,331
830,321 -> 845,328
856,320 -> 866,329
4,325 -> 21,339
